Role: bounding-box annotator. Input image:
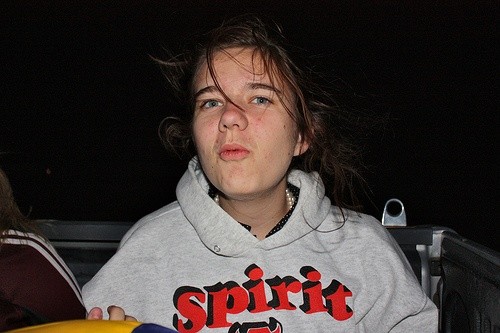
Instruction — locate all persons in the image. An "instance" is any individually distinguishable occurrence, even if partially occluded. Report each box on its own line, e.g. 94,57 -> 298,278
82,17 -> 438,333
0,170 -> 88,332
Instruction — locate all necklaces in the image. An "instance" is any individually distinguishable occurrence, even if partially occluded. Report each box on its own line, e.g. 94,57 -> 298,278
214,188 -> 296,210
